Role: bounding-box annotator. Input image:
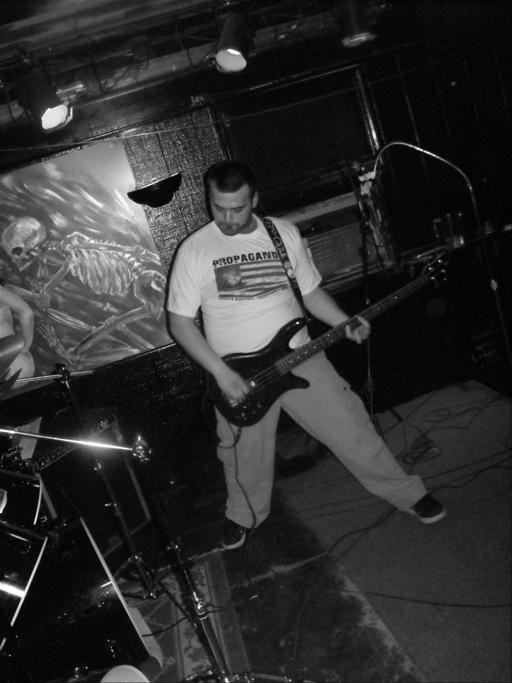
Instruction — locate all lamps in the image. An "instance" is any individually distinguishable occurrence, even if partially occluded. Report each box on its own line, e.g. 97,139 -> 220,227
10,61 -> 75,131
212,30 -> 248,72
127,171 -> 184,208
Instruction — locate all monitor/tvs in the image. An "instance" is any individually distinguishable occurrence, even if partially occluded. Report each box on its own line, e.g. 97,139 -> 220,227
208,61 -> 392,217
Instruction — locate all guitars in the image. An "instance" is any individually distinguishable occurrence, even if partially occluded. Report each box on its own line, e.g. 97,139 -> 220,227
203,251 -> 460,428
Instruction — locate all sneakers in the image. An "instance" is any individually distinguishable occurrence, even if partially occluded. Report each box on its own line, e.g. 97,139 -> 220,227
220,516 -> 247,550
404,492 -> 445,525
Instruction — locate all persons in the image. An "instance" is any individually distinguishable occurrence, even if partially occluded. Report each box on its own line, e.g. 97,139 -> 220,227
164,161 -> 448,550
0,285 -> 122,549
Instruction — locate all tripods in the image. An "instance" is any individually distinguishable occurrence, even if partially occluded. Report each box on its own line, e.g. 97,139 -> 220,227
1,365 -> 305,683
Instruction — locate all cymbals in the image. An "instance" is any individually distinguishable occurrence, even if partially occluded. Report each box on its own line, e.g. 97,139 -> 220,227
0,333 -> 26,376
